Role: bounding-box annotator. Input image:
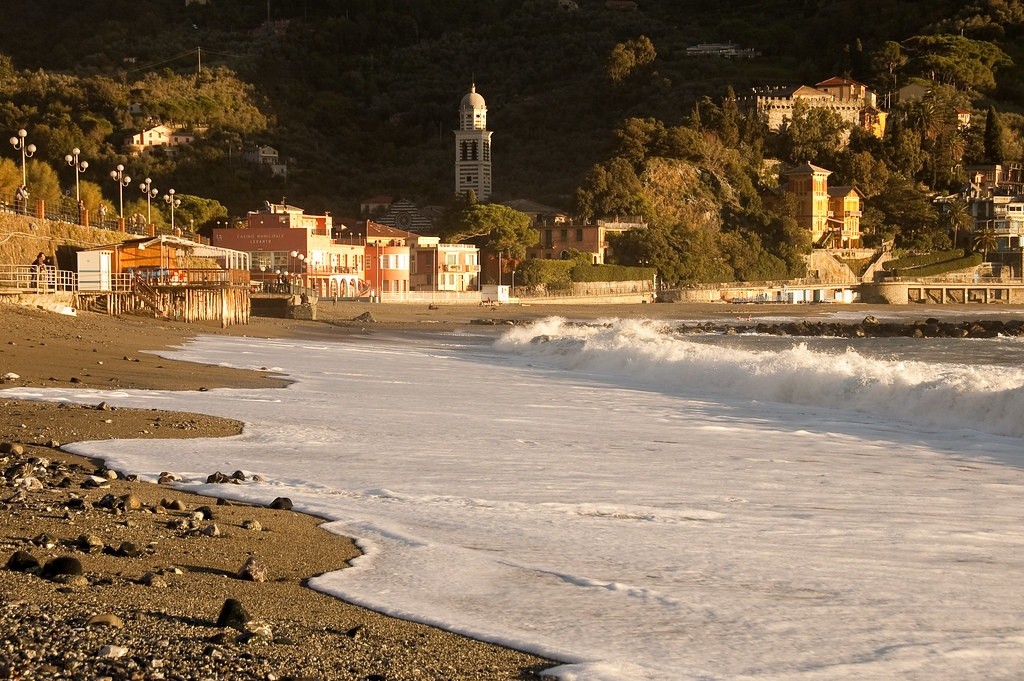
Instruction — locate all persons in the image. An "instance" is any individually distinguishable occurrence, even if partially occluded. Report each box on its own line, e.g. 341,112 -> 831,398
128,212 -> 147,234
249,285 -> 308,306
78,199 -> 85,225
136,266 -> 142,282
184,270 -> 187,284
332,293 -> 338,306
31,251 -> 46,288
98,203 -> 107,225
14,184 -> 30,214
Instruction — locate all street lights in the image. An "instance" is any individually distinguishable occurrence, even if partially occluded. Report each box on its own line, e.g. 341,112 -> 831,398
372,243 -> 380,304
290,250 -> 297,273
298,254 -> 304,273
139,177 -> 159,224
9,129 -> 36,214
310,260 -> 315,298
110,164 -> 132,218
64,148 -> 89,203
163,188 -> 181,229
260,267 -> 265,282
